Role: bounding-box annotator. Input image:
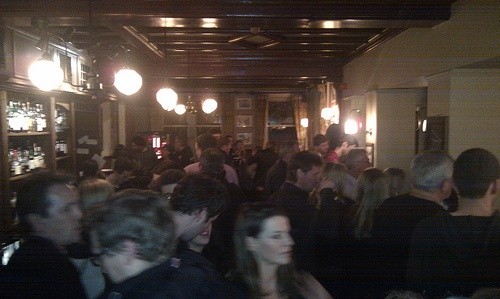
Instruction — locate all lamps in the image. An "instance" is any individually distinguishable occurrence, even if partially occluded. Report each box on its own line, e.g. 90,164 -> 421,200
201,99 -> 218,115
63,26 -> 75,42
113,63 -> 144,97
35,35 -> 48,51
155,32 -> 179,112
343,118 -> 373,136
28,51 -> 65,92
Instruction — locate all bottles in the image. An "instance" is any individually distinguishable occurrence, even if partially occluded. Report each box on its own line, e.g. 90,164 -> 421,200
10,192 -> 19,224
5,100 -> 47,132
55,105 -> 69,157
8,140 -> 46,176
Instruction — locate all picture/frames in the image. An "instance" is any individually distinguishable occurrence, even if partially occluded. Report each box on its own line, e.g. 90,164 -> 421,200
364,143 -> 374,167
235,130 -> 254,146
236,113 -> 255,129
234,95 -> 254,112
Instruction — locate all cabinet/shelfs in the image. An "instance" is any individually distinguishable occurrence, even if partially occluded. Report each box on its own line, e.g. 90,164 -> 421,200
0,83 -> 55,248
53,91 -> 76,178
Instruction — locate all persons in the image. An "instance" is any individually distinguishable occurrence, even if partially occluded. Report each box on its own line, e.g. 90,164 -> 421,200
0,124 -> 500,299
